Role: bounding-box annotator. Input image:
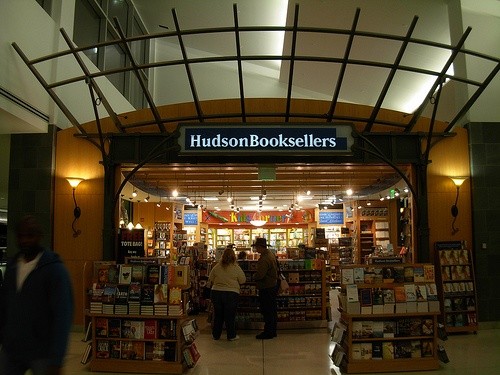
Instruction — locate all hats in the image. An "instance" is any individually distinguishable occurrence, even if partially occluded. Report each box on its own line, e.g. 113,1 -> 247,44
249,237 -> 272,248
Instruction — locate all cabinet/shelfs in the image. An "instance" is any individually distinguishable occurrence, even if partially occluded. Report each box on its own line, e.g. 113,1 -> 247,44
81,199 -> 478,375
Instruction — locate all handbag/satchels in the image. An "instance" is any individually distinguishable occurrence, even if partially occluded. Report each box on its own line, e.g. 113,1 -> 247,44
277,278 -> 290,297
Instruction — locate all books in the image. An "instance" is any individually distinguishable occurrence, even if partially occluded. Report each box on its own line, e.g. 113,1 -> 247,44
81,262 -> 200,368
328,266 -> 437,367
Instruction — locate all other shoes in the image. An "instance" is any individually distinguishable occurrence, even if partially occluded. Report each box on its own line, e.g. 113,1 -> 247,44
256,333 -> 273,340
227,334 -> 240,341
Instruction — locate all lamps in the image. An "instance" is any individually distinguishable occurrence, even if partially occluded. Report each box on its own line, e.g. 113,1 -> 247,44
448,176 -> 468,235
133,173 -> 399,212
63,177 -> 83,237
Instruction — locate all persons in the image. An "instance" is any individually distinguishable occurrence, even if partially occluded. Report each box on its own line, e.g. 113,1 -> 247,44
253,238 -> 280,340
207,249 -> 246,341
0,217 -> 75,375
